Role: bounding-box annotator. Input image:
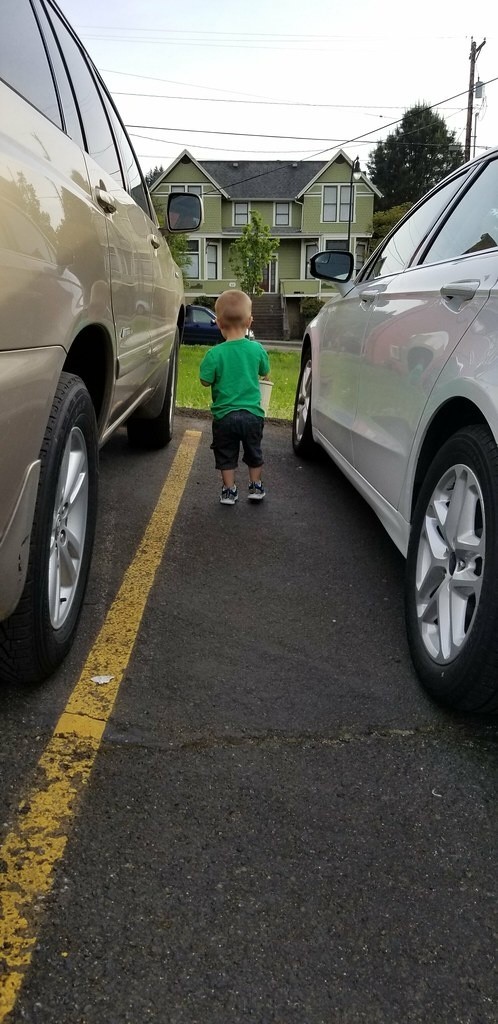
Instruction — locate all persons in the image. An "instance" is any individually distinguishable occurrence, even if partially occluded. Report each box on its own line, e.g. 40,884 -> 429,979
198,290 -> 272,504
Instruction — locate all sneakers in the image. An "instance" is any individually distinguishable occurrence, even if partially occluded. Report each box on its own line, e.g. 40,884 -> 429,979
247,480 -> 266,499
219,483 -> 238,505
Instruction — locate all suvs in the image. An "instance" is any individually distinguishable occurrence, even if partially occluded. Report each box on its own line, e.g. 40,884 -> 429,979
0,2 -> 187,683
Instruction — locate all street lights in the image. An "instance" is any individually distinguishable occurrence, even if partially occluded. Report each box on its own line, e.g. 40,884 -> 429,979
347,156 -> 363,251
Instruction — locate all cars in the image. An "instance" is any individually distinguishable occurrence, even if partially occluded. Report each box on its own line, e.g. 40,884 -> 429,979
182,303 -> 255,346
291,148 -> 498,713
